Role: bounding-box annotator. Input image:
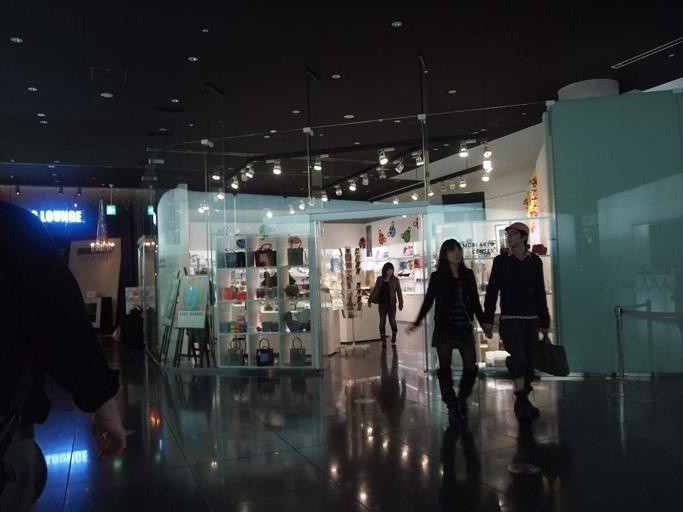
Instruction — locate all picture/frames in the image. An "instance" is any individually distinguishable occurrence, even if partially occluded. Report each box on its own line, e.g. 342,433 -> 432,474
495,225 -> 512,254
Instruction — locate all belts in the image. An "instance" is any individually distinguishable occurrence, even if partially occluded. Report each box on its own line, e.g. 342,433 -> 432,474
5,423 -> 33,444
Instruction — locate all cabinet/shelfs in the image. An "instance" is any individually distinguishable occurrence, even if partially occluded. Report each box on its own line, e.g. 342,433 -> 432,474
372,253 -> 432,293
462,254 -> 556,362
214,233 -> 319,372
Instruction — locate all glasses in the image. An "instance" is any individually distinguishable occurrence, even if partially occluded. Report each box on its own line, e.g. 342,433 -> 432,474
505,232 -> 516,237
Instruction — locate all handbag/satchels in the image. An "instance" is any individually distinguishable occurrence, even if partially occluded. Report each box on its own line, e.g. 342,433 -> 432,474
225,236 -> 310,366
535,341 -> 569,376
372,288 -> 380,304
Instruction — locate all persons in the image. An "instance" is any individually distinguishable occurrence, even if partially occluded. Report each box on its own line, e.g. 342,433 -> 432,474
366,262 -> 404,348
408,238 -> 484,416
0,201 -> 127,510
261,272 -> 271,284
485,223 -> 550,420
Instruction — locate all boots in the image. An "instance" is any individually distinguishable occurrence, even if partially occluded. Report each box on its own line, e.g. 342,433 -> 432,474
459,366 -> 477,416
437,369 -> 460,420
379,328 -> 387,349
391,325 -> 397,343
514,386 -> 539,419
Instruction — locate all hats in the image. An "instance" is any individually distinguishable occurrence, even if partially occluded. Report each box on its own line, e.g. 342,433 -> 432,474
505,223 -> 529,234
